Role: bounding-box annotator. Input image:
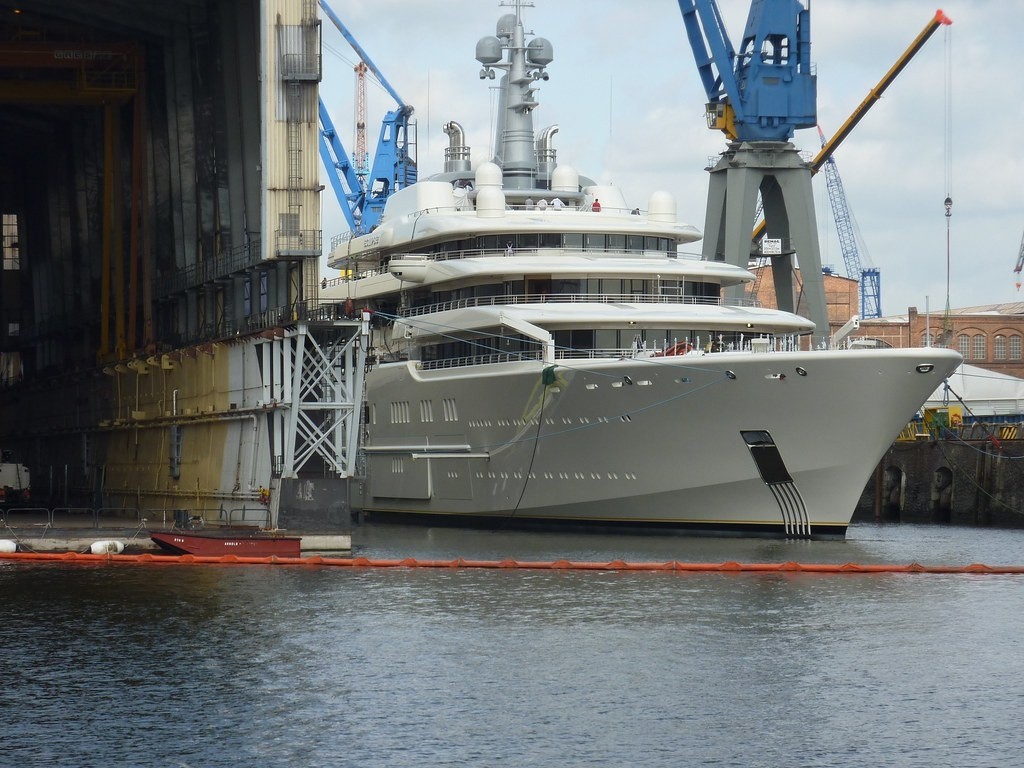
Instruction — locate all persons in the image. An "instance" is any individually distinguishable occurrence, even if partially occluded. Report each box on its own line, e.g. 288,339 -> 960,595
536,196 -> 547,211
524,196 -> 533,210
631,208 -> 640,215
550,196 -> 566,211
933,467 -> 952,524
320,278 -> 327,289
882,465 -> 901,522
591,199 -> 601,212
505,240 -> 513,252
705,340 -> 715,353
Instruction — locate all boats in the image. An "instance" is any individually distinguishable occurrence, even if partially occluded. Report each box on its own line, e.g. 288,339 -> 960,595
1,1 -> 321,564
320,1 -> 964,542
